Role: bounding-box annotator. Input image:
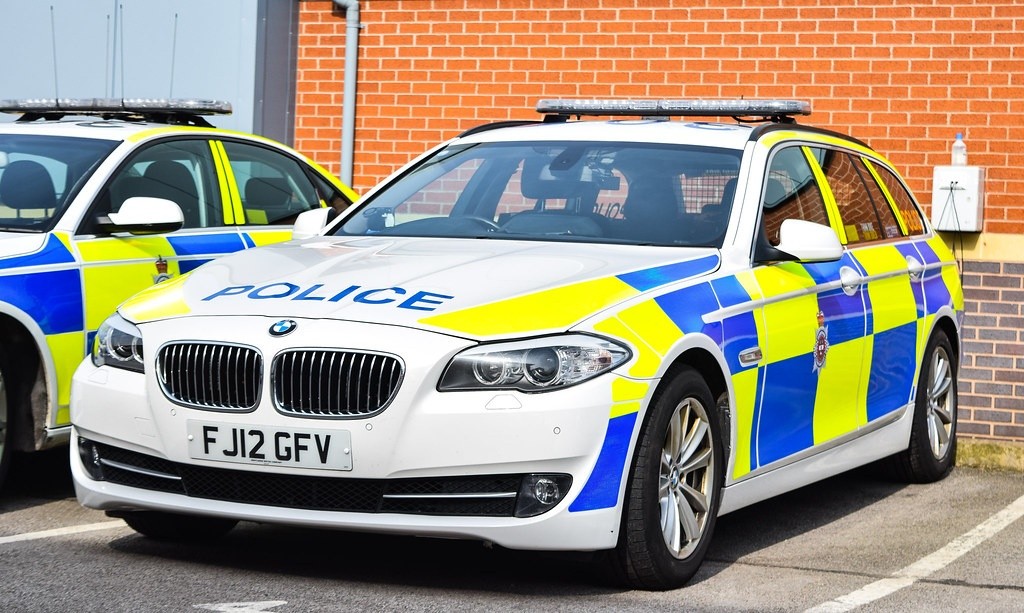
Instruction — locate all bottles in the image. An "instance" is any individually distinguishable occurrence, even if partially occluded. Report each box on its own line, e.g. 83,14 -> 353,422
951,132 -> 968,166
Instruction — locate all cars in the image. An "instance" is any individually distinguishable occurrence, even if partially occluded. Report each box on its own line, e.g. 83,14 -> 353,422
68,96 -> 967,591
0,98 -> 378,479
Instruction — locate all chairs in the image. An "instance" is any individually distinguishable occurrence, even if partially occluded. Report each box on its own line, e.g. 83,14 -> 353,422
0,160 -> 296,234
498,151 -> 788,243
